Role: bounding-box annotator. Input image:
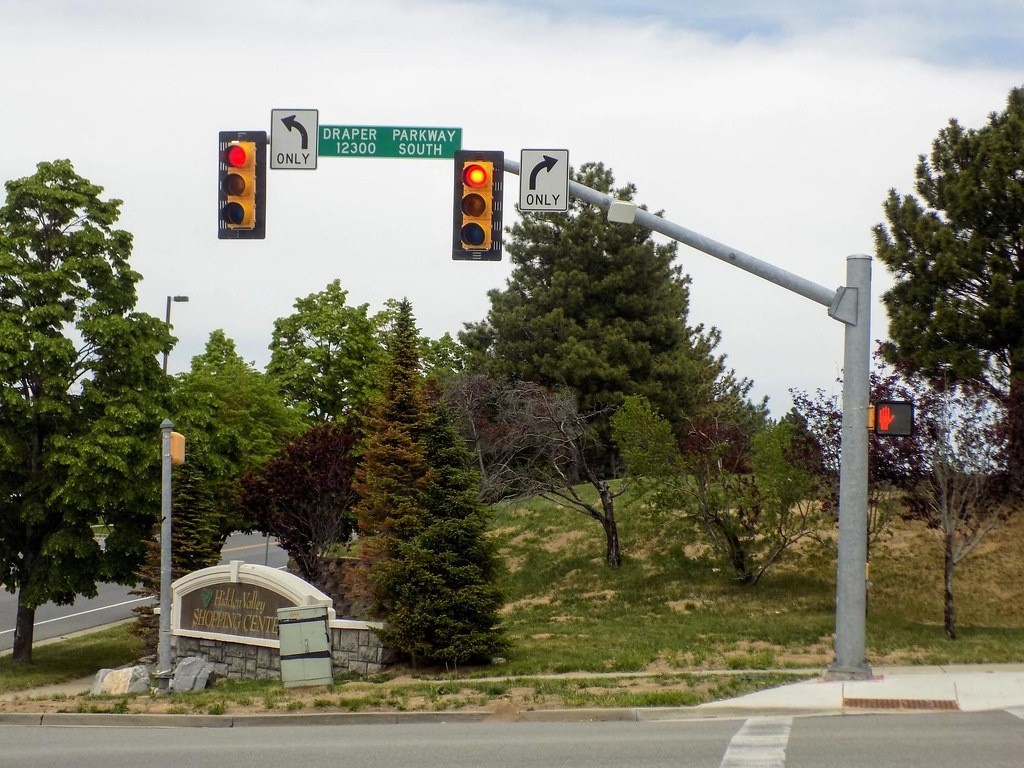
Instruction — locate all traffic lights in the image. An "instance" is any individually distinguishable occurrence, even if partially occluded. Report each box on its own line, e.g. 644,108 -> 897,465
875,400 -> 916,436
450,149 -> 505,261
217,131 -> 267,240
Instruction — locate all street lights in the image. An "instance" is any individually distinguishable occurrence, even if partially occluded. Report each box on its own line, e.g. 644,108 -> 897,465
161,294 -> 191,373
